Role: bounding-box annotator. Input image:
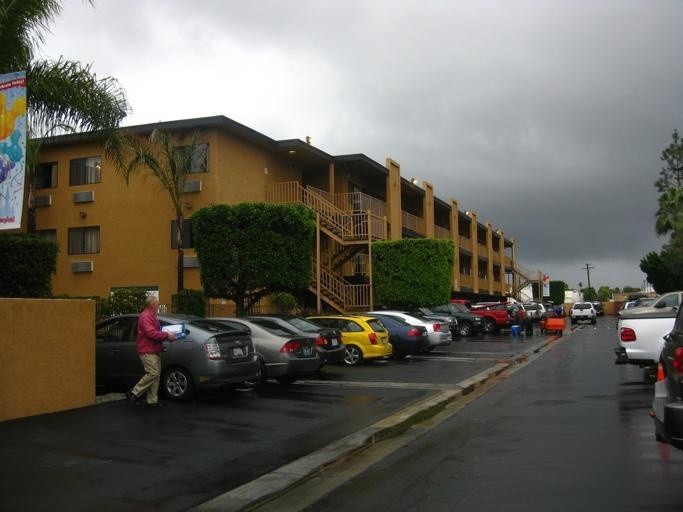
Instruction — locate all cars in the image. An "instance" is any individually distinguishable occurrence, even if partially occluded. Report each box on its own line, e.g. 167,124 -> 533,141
362,314 -> 427,362
400,299 -> 565,359
369,309 -> 453,352
268,312 -> 344,373
613,290 -> 683,368
96,315 -> 256,398
303,315 -> 394,365
209,314 -> 320,386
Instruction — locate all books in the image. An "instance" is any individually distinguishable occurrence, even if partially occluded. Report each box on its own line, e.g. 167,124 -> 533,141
162,321 -> 186,342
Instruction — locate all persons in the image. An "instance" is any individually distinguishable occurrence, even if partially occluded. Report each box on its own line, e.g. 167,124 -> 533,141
124,294 -> 176,409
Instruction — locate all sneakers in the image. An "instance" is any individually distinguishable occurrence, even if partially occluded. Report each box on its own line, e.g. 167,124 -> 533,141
126,390 -> 140,407
146,402 -> 163,409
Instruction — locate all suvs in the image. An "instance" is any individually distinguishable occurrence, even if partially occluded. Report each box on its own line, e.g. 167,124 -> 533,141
570,302 -> 597,325
649,294 -> 682,453
590,302 -> 603,316
